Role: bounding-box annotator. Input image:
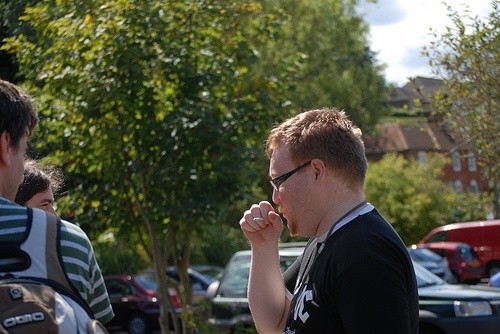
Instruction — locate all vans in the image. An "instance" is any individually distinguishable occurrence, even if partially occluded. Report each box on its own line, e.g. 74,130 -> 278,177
418,220 -> 500,277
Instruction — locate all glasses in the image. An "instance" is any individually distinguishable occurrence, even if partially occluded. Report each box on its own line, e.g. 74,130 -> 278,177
269,160 -> 311,192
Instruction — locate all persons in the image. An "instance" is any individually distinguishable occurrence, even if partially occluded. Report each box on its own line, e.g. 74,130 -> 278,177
15,160 -> 64,218
0,80 -> 115,324
240,107 -> 419,334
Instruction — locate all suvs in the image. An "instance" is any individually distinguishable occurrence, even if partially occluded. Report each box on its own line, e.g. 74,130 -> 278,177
207,240 -> 500,334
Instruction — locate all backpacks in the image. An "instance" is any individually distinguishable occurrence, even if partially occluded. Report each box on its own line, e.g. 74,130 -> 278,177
0,205 -> 110,334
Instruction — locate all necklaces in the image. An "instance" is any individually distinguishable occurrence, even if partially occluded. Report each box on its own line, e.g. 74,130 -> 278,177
284,200 -> 367,334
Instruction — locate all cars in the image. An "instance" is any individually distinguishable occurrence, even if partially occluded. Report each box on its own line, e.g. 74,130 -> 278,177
405,242 -> 486,285
100,264 -> 248,334
